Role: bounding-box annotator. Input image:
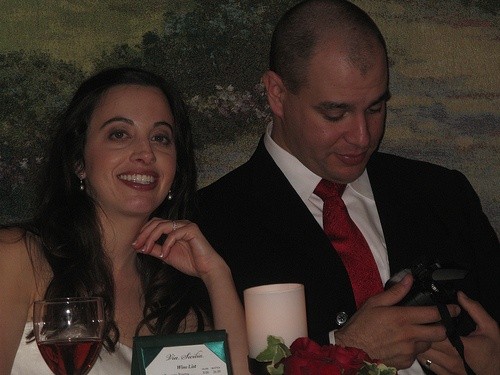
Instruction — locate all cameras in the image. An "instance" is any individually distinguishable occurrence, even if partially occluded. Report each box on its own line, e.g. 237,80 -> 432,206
384,259 -> 478,337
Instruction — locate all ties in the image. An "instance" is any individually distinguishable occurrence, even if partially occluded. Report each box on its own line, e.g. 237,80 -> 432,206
313,180 -> 384,312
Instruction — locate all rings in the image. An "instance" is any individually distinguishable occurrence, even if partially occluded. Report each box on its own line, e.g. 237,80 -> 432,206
173,221 -> 176,231
423,360 -> 431,368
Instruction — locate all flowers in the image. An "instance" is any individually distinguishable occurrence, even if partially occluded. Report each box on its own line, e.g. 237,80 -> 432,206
255,335 -> 397,375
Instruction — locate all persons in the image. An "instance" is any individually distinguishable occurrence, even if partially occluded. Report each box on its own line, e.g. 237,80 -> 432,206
180,0 -> 500,375
0,66 -> 253,375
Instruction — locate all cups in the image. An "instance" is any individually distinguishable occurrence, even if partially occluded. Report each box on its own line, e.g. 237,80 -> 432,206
32,297 -> 106,375
